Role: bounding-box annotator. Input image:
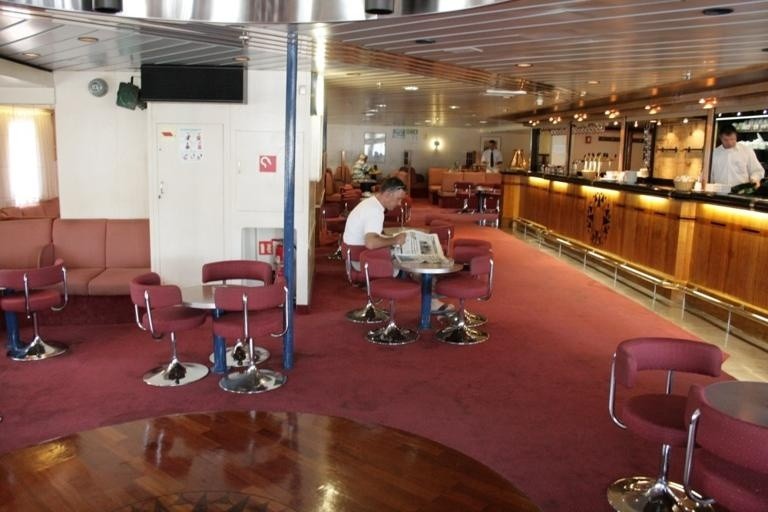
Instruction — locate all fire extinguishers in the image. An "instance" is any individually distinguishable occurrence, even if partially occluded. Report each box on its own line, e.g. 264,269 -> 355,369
271,238 -> 285,276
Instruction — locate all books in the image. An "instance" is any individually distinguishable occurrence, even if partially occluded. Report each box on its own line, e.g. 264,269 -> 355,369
391,230 -> 455,272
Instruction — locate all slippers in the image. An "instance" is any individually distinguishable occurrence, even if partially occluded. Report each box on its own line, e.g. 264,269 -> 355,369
428,300 -> 457,314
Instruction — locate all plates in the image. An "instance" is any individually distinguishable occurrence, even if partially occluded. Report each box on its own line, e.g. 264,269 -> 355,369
707,183 -> 733,197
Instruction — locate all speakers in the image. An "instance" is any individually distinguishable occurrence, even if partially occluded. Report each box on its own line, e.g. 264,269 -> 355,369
141,63 -> 247,101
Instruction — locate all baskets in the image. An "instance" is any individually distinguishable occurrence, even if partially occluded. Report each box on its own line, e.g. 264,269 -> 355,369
671,177 -> 695,191
582,168 -> 598,180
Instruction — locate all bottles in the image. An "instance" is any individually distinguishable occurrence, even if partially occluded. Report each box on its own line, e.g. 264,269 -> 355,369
732,118 -> 768,133
540,161 -> 578,177
582,151 -> 618,171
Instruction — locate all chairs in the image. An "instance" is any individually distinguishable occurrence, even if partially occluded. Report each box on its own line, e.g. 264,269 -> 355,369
682,389 -> 768,511
201,260 -> 274,368
317,162 -> 504,348
0,258 -> 70,362
129,272 -> 210,389
213,275 -> 291,395
603,336 -> 724,512
0,197 -> 152,296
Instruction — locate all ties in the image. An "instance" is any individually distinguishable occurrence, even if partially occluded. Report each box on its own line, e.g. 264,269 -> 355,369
489,151 -> 495,168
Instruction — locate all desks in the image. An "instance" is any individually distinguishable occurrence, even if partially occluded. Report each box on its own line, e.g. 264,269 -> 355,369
181,276 -> 267,377
0,408 -> 539,508
702,381 -> 768,427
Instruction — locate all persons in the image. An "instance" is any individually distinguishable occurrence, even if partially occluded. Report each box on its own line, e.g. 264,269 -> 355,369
350,153 -> 378,187
339,175 -> 455,323
481,139 -> 504,174
372,164 -> 383,180
708,121 -> 767,195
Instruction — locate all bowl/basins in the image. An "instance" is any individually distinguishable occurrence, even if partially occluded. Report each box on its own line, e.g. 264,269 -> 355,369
674,181 -> 695,196
579,169 -> 649,185
